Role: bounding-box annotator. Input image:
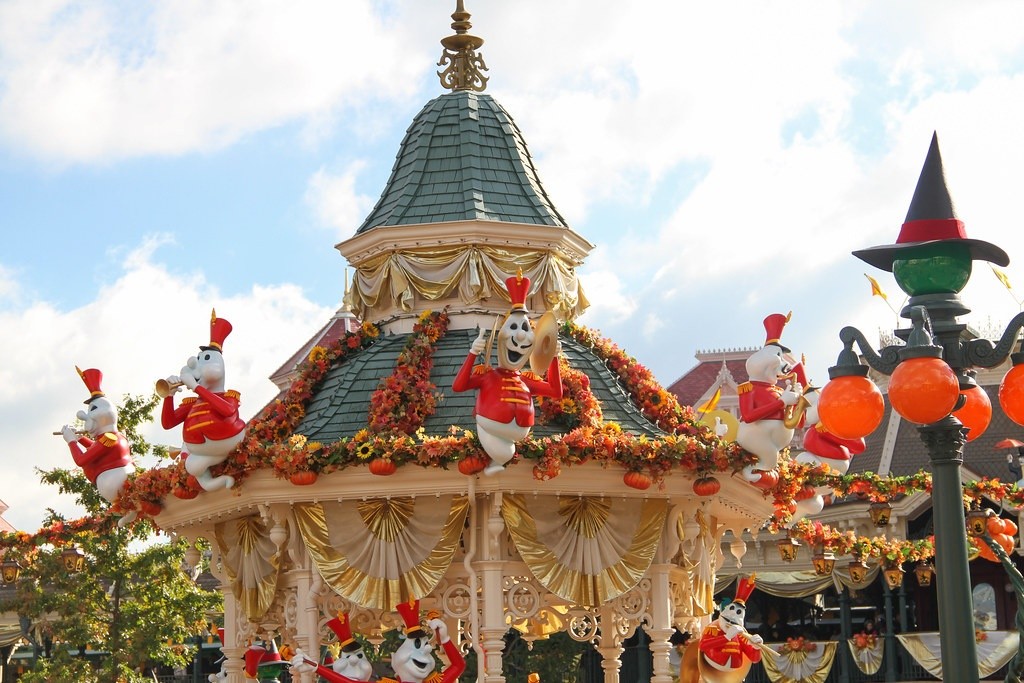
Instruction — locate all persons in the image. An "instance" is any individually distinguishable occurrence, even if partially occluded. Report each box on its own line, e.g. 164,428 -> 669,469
720,585 -> 924,645
32,654 -> 196,681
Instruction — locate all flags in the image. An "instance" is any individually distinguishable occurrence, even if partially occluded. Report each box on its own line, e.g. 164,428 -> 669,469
697,389 -> 720,414
992,268 -> 1012,289
866,275 -> 886,299
